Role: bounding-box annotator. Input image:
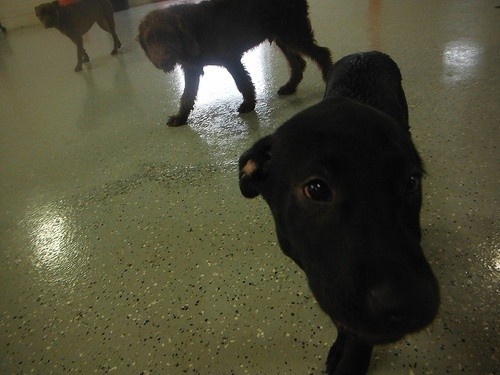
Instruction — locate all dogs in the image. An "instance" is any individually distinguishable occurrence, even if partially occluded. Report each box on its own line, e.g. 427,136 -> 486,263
137,1 -> 333,127
34,1 -> 122,71
238,50 -> 441,375
